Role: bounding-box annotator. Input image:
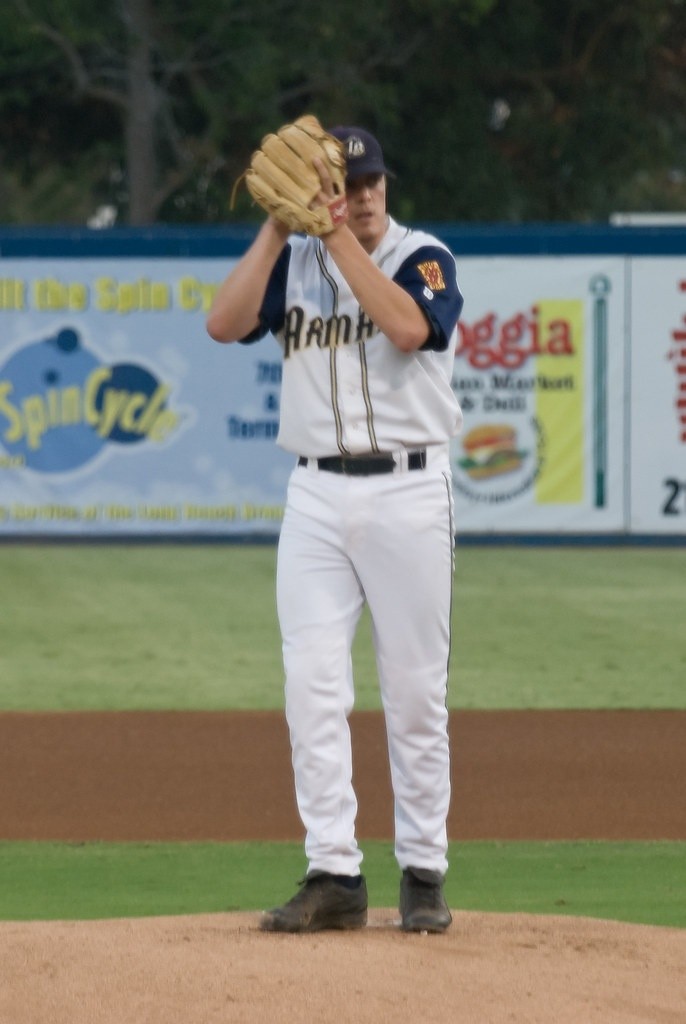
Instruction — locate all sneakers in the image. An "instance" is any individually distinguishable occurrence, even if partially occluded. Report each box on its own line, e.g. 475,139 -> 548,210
397,866 -> 451,932
261,870 -> 368,931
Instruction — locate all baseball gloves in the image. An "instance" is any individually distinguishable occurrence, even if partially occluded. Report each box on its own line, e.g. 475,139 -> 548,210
243,114 -> 350,237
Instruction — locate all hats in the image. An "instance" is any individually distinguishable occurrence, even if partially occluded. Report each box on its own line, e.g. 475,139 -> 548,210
325,125 -> 389,176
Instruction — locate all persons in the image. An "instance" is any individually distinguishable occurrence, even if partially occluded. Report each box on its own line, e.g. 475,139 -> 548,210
206,116 -> 466,932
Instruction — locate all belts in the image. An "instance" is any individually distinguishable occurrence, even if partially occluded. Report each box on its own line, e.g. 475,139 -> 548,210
298,452 -> 426,477
